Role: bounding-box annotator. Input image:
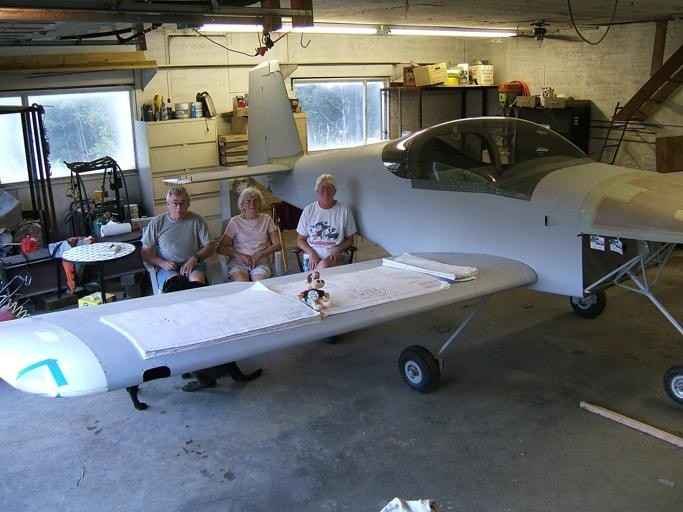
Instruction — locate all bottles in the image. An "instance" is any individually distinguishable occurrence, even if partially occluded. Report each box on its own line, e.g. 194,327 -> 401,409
146,95 -> 175,121
190,104 -> 196,118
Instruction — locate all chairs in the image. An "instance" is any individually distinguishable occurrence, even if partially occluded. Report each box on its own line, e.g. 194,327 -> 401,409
143,204 -> 358,295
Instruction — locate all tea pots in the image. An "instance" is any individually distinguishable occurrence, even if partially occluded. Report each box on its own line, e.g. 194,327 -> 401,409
90,216 -> 110,237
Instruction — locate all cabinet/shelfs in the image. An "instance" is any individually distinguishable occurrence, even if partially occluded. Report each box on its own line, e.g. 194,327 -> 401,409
219,165 -> 258,220
514,103 -> 591,159
231,113 -> 308,211
133,116 -> 223,241
0,229 -> 146,305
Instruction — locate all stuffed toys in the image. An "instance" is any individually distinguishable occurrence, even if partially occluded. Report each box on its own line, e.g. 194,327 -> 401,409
296,270 -> 332,312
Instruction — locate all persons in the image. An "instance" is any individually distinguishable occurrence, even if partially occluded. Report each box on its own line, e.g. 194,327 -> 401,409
214,185 -> 283,281
139,182 -> 218,293
293,171 -> 358,345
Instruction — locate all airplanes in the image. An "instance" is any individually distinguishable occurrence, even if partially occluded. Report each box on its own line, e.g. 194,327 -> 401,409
0,59 -> 683,404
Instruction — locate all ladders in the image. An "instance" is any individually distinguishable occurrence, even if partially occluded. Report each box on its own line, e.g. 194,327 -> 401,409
598,101 -> 631,165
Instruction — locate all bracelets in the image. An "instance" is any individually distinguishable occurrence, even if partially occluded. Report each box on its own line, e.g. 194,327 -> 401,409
194,253 -> 202,264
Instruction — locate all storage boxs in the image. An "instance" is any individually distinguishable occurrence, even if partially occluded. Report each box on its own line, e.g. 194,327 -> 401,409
218,132 -> 248,166
468,64 -> 494,86
412,62 -> 449,87
403,66 -> 417,87
77,291 -> 117,308
540,97 -> 566,109
516,95 -> 540,108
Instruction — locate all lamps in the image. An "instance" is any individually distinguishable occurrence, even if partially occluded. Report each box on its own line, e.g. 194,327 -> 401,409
177,20 -> 386,36
384,24 -> 520,37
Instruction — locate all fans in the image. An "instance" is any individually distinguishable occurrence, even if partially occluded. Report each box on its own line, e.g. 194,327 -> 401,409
511,19 -> 584,48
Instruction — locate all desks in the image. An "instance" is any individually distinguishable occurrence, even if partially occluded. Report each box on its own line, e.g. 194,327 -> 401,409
62,241 -> 136,304
